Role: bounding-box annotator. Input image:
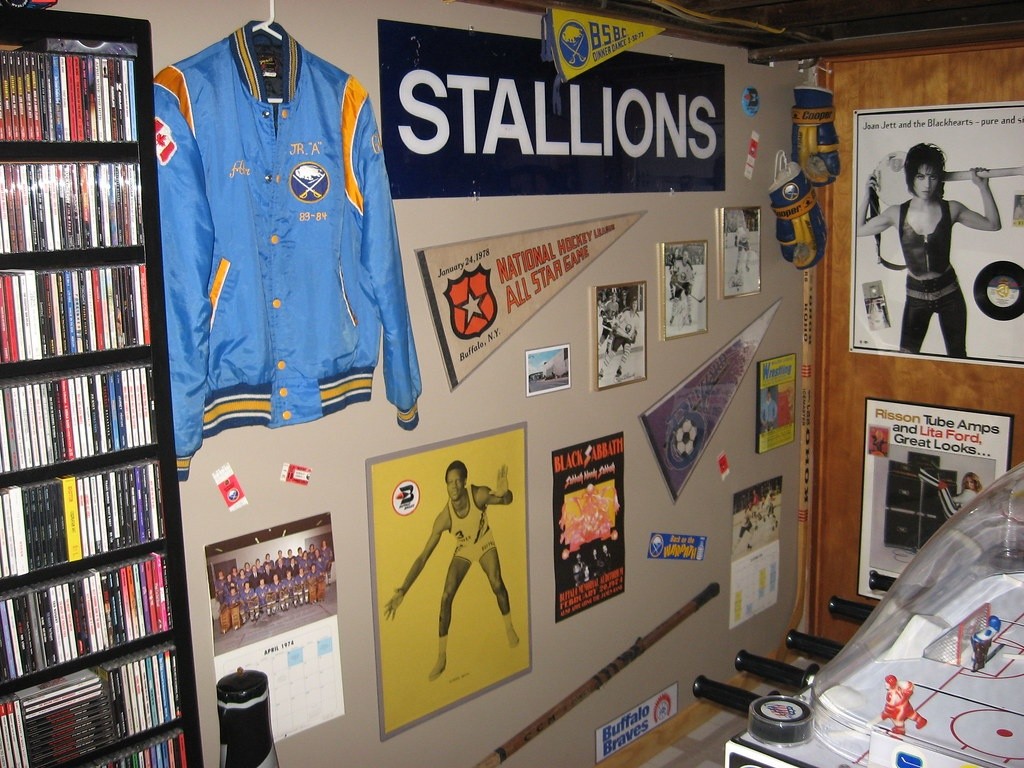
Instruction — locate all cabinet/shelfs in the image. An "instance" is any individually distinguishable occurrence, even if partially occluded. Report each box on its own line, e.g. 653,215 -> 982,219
0,3 -> 204,767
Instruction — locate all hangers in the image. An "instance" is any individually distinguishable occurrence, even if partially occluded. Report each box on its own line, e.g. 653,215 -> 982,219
247,0 -> 289,103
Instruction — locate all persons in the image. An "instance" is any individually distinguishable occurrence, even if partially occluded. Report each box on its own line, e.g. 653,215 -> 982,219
950,471 -> 983,506
212,540 -> 333,635
383,461 -> 519,683
759,390 -> 779,435
881,675 -> 926,738
856,143 -> 1001,359
527,208 -> 764,386
971,615 -> 1001,672
730,494 -> 778,557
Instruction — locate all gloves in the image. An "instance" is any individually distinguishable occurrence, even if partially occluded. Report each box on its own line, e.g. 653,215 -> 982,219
768,162 -> 827,270
790,86 -> 840,187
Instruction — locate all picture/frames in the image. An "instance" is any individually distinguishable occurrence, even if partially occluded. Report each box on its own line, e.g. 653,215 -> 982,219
593,280 -> 648,391
718,206 -> 762,299
662,240 -> 709,341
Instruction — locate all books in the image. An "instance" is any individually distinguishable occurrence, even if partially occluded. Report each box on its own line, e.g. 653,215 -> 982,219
0,52 -> 196,768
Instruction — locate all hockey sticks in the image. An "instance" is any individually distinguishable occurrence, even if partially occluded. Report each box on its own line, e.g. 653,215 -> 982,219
674,283 -> 705,303
